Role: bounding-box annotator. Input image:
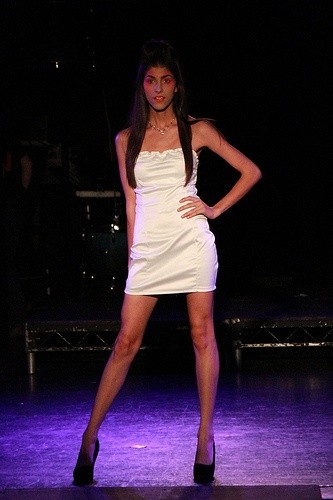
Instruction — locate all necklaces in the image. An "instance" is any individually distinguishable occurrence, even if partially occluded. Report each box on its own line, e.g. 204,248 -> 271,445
147,116 -> 177,134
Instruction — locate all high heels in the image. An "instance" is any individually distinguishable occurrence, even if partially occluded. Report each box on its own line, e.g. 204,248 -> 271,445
193,441 -> 215,485
72,438 -> 100,487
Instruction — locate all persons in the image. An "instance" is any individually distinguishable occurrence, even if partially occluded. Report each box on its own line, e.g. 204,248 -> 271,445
73,51 -> 262,484
0,125 -> 33,290
